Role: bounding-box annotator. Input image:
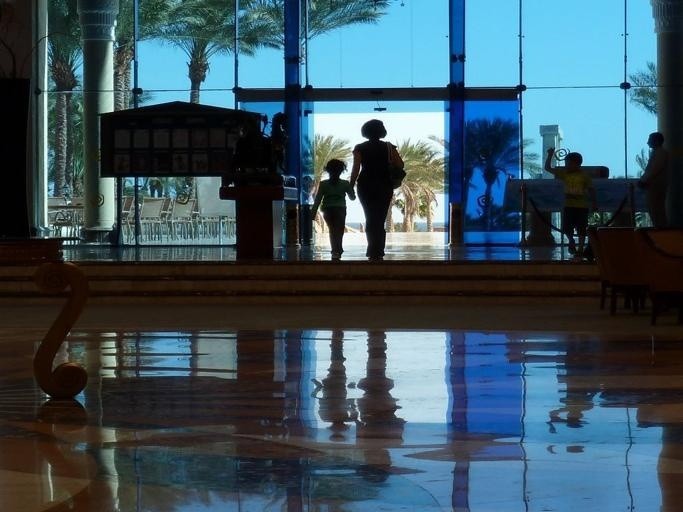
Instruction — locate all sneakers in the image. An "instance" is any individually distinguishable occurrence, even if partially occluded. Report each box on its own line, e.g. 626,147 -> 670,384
333,250 -> 342,260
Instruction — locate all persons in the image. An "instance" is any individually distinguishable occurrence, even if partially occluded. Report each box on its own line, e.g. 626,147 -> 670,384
311,159 -> 354,258
638,133 -> 668,229
348,121 -> 404,260
543,148 -> 597,256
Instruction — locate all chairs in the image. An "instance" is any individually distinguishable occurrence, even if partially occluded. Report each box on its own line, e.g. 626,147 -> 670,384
123,197 -> 137,242
638,225 -> 683,326
591,226 -> 640,316
157,198 -> 174,239
221,216 -> 238,240
168,197 -> 196,236
196,214 -> 220,238
132,197 -> 165,239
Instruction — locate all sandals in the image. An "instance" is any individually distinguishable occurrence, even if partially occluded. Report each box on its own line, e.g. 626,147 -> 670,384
568,239 -> 576,253
367,253 -> 385,262
572,251 -> 585,265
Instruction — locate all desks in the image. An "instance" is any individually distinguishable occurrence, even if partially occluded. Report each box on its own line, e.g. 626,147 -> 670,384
0,235 -> 90,399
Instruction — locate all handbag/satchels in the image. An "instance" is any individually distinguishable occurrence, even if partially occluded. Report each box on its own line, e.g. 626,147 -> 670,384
387,142 -> 403,188
